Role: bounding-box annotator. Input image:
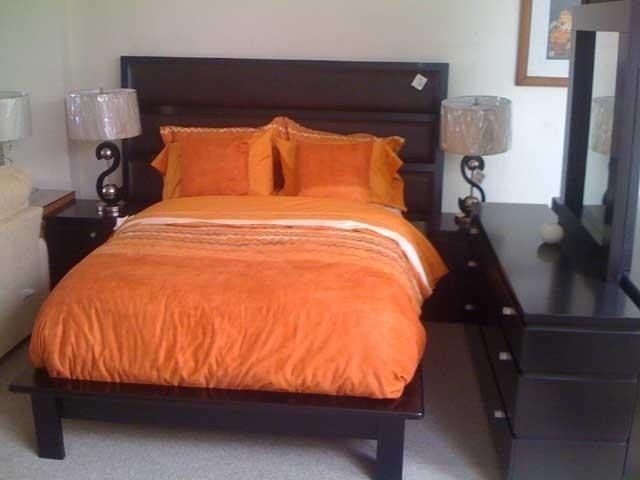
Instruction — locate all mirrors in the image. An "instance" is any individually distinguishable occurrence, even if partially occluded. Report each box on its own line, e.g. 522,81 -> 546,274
550,0 -> 640,282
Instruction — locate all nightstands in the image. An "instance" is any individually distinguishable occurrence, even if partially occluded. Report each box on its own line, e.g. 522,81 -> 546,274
423,213 -> 469,324
45,198 -> 117,292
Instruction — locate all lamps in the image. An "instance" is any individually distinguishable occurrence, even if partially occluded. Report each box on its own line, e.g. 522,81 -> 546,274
64,86 -> 145,212
589,96 -> 614,224
439,96 -> 512,234
0,90 -> 33,223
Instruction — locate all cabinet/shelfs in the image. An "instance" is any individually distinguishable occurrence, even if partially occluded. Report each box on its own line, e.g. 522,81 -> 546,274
454,202 -> 640,478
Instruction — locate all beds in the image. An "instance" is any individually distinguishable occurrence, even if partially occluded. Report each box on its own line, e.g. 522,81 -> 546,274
32,55 -> 450,479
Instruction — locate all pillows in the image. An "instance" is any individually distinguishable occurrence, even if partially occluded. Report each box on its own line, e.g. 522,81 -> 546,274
151,117 -> 408,211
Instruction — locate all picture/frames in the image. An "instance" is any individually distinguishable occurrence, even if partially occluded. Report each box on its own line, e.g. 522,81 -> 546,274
515,1 -> 572,88
